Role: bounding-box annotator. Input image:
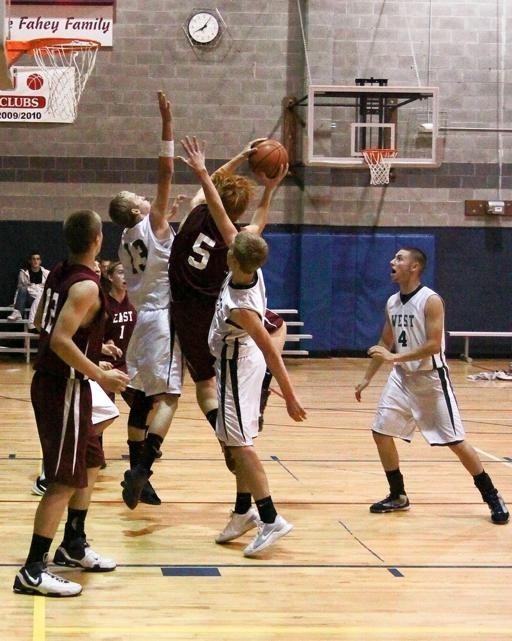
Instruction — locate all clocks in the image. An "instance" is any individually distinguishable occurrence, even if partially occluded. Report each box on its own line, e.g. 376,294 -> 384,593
183,7 -> 227,50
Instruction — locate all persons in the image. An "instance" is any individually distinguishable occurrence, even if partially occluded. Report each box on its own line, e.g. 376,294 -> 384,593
355,247 -> 509,524
171,137 -> 289,474
107,89 -> 188,509
33,257 -> 101,496
27,292 -> 44,334
7,253 -> 51,320
12,210 -> 130,597
173,134 -> 307,557
100,262 -> 162,473
100,260 -> 110,276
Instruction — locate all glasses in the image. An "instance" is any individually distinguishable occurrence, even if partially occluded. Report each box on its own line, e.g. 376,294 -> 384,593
31,257 -> 41,260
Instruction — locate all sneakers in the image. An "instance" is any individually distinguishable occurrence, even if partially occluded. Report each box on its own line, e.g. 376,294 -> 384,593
8,309 -> 21,320
371,487 -> 413,513
215,510 -> 256,543
52,540 -> 117,573
483,491 -> 510,524
14,568 -> 84,598
122,465 -> 148,509
242,514 -> 294,557
140,469 -> 163,505
33,476 -> 48,496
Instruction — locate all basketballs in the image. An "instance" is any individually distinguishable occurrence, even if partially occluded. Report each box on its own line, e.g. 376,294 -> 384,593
248,138 -> 289,179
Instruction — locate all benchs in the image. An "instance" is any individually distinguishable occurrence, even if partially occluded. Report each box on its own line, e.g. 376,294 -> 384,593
448,332 -> 512,363
0,305 -> 313,356
